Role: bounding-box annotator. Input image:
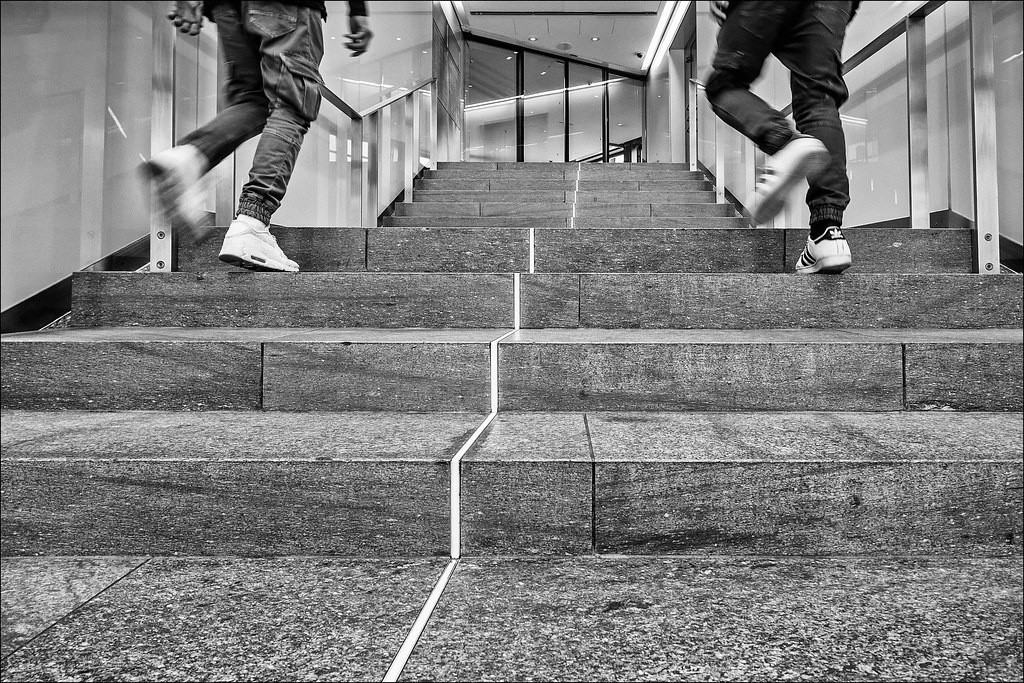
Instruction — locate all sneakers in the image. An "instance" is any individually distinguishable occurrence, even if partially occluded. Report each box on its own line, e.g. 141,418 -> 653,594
140,148 -> 208,241
748,133 -> 829,225
218,219 -> 299,272
795,226 -> 853,274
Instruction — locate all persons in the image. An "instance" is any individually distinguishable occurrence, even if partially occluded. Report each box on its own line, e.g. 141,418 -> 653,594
139,1 -> 374,272
703,1 -> 860,274
